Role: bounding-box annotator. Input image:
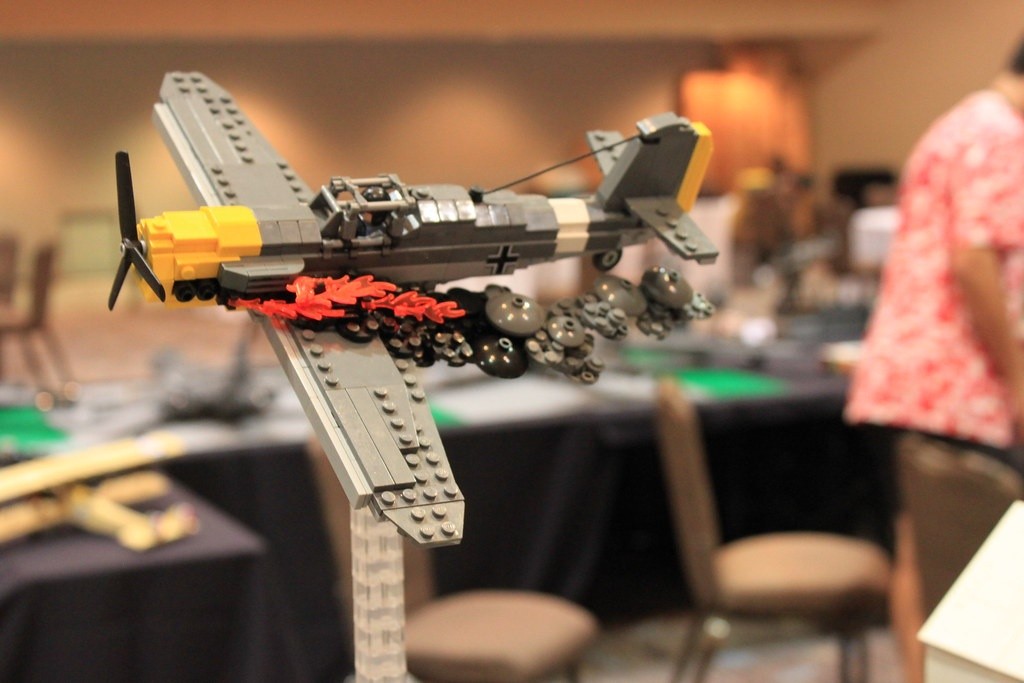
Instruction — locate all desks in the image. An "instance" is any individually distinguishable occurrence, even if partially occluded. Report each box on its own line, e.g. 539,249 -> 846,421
0,309 -> 902,683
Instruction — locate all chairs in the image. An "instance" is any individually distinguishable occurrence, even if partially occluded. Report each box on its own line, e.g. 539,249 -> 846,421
653,374 -> 892,683
307,426 -> 599,683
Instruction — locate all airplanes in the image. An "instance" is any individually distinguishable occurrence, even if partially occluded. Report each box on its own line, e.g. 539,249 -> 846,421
108,70 -> 720,550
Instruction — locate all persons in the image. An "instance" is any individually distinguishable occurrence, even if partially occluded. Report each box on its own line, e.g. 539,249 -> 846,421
841,40 -> 1024,683
